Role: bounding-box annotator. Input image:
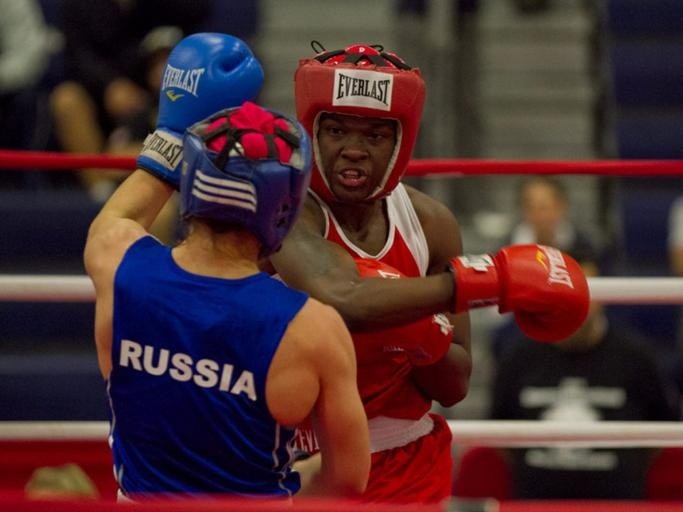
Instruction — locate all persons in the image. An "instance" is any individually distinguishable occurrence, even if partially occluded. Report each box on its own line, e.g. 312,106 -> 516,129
83,32 -> 371,503
486,250 -> 675,498
0,0 -> 184,205
665,196 -> 683,276
270,40 -> 590,511
484,176 -> 611,357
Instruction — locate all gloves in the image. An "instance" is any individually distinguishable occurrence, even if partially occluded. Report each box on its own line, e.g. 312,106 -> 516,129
345,256 -> 453,368
132,28 -> 267,193
440,240 -> 591,346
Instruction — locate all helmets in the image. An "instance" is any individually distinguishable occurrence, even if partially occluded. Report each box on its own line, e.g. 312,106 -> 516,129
290,39 -> 425,213
178,98 -> 315,256
